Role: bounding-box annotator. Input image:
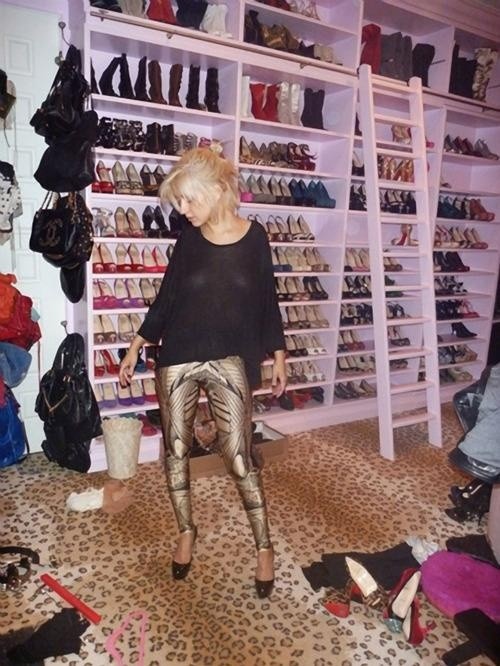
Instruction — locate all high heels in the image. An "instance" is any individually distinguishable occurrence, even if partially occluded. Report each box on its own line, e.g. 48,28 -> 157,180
246,175 -> 265,203
355,112 -> 363,138
90,1 -> 121,12
134,347 -> 147,372
142,245 -> 159,272
305,306 -> 321,328
100,315 -> 119,345
153,279 -> 161,296
127,242 -> 144,273
287,216 -> 306,242
433,251 -> 470,272
94,383 -> 104,411
129,314 -> 142,338
115,243 -> 133,273
256,214 -> 273,242
439,367 -> 473,386
185,133 -> 198,150
277,276 -> 292,300
392,124 -> 434,148
444,134 -> 499,160
139,164 -> 158,196
303,248 -> 318,272
300,144 -> 317,171
115,207 -> 136,237
102,383 -> 116,409
255,543 -> 275,599
126,164 -> 144,195
313,276 -> 329,300
297,216 -> 315,242
248,214 -> 256,220
267,176 -> 287,204
113,278 -> 132,308
252,387 -> 323,413
342,276 -> 405,299
249,141 -> 264,165
275,285 -> 283,300
334,380 -> 377,400
172,526 -> 197,580
348,183 -> 416,216
312,248 -> 330,271
434,276 -> 468,296
93,280 -> 108,309
163,125 -> 176,155
309,181 -> 329,207
299,362 -> 316,382
293,277 -> 311,300
284,336 -> 301,357
146,0 -> 179,26
138,414 -> 158,437
435,224 -> 488,248
92,243 -> 106,274
212,139 -> 226,160
285,277 -> 303,301
128,120 -> 149,152
118,349 -> 130,361
294,306 -> 310,329
351,150 -> 429,184
288,142 -> 307,170
286,307 -> 300,329
266,216 -> 285,242
384,568 -> 422,632
102,209 -> 117,237
303,277 -> 321,301
152,246 -> 169,273
118,314 -> 134,343
94,349 -> 105,376
238,169 -> 254,202
100,349 -> 121,375
201,5 -> 234,40
92,170 -> 100,193
240,136 -> 253,163
290,362 -> 307,383
96,161 -> 114,193
259,143 -> 275,167
154,206 -> 178,239
255,0 -> 321,22
173,132 -> 187,157
270,246 -> 283,272
138,279 -> 156,305
112,162 -> 130,194
344,555 -> 387,613
451,323 -> 477,338
338,331 -> 364,352
440,173 -> 452,189
340,303 -> 408,327
130,380 -> 146,406
278,143 -> 299,169
198,137 -> 211,150
98,242 -> 117,273
279,306 -> 290,329
317,181 -> 336,208
92,139 -> 101,148
310,335 -> 328,354
338,357 -> 376,376
444,478 -> 492,526
307,361 -> 325,381
126,207 -> 146,237
116,382 -> 132,406
385,327 -> 410,348
435,299 -> 479,321
294,248 -> 312,272
278,178 -> 296,205
437,195 -> 495,222
124,278 -> 146,307
289,179 -> 306,206
142,205 -> 162,238
145,123 -> 162,155
93,315 -> 106,344
89,208 -> 104,237
402,596 -> 425,646
298,180 -> 316,206
142,379 -> 159,404
257,176 -> 276,204
276,216 -> 293,242
345,247 -> 403,271
284,247 -> 301,272
113,119 -> 133,151
275,247 -> 293,272
269,141 -> 287,168
313,305 -> 329,329
291,335 -> 308,356
98,117 -> 115,149
97,279 -> 123,309
119,0 -> 147,20
391,222 -> 418,247
153,165 -> 167,185
166,243 -> 175,260
438,345 -> 477,366
245,9 -> 342,66
283,362 -> 299,384
300,335 -> 318,355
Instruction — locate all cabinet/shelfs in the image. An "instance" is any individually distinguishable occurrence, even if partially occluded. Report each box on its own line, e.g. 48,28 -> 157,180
72,0 -> 500,473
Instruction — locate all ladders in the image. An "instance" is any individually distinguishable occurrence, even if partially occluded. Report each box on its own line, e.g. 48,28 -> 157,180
359,64 -> 441,459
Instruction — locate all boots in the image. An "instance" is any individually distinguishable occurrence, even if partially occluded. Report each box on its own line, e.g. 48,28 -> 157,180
99,57 -> 121,96
134,56 -> 150,102
450,43 -> 496,101
118,53 -> 136,99
361,24 -> 436,87
249,83 -> 268,120
263,85 -> 281,124
176,0 -> 207,34
204,68 -> 221,115
276,81 -> 290,124
148,60 -> 167,105
185,65 -> 205,111
242,76 -> 254,119
91,57 -> 100,95
169,63 -> 183,107
301,88 -> 326,131
290,84 -> 302,126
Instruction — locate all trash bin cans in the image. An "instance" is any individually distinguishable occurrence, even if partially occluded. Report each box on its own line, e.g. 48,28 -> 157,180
101,417 -> 143,479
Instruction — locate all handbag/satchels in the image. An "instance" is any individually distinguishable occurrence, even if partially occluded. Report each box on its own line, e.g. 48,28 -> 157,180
30,44 -> 91,137
33,111 -> 98,192
29,189 -> 82,255
34,333 -> 104,446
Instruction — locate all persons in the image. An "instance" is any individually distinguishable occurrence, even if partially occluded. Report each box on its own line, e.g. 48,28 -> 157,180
121,149 -> 287,601
